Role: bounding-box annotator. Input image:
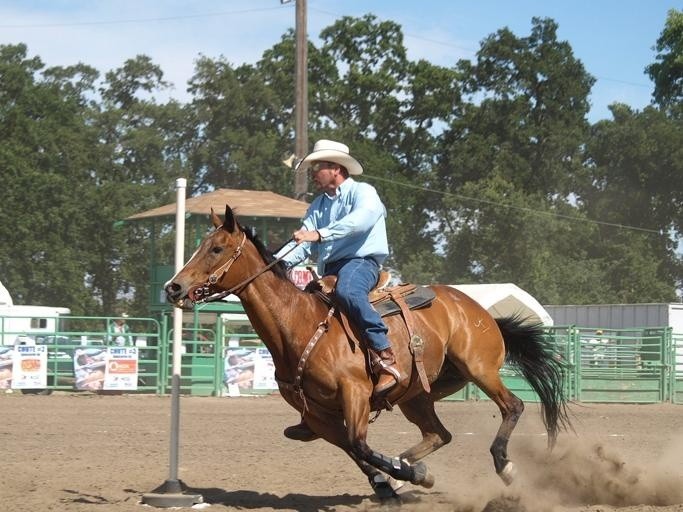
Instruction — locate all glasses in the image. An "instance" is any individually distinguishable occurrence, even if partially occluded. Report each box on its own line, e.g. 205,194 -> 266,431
312,162 -> 341,171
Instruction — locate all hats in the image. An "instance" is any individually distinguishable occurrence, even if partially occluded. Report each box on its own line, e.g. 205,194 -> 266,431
294,139 -> 363,176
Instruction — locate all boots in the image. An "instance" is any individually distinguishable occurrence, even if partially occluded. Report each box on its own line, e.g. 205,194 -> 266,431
284,419 -> 313,440
374,348 -> 408,394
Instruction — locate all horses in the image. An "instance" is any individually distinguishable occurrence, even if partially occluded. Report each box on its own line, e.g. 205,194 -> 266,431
162,203 -> 591,508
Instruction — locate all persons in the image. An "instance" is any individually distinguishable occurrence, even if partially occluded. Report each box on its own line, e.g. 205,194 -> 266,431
274,138 -> 396,441
103,313 -> 134,348
589,330 -> 609,365
